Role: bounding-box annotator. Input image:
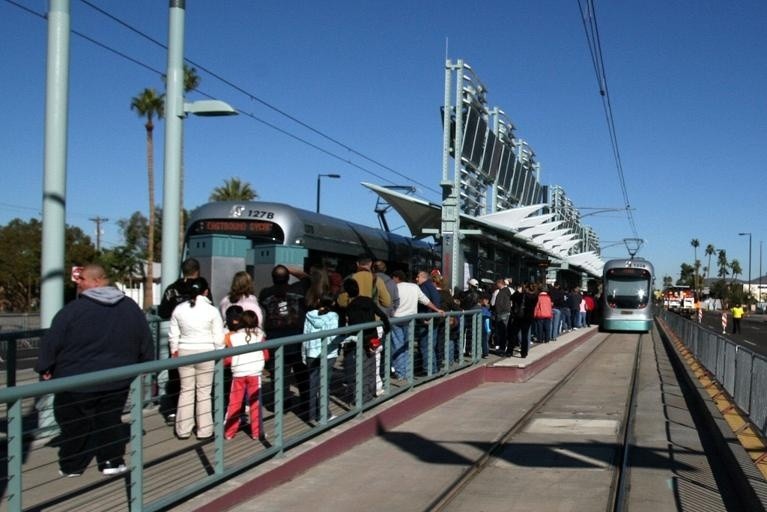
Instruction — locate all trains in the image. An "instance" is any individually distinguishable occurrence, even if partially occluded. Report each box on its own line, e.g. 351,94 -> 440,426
177,200 -> 543,308
597,256 -> 657,333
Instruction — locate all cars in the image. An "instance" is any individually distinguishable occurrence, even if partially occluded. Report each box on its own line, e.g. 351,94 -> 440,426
668,300 -> 680,310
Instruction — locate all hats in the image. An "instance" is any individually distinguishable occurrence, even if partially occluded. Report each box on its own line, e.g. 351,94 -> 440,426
522,282 -> 538,294
537,284 -> 552,292
467,278 -> 478,287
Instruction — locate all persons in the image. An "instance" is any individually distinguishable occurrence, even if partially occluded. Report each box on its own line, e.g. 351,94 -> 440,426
728,301 -> 744,334
155,256 -> 224,442
219,270 -> 269,440
32,263 -> 156,478
257,265 -> 314,413
481,278 -> 604,358
300,256 -> 488,421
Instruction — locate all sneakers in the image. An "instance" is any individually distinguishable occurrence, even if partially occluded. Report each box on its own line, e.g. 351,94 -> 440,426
326,415 -> 338,422
166,414 -> 176,425
499,350 -> 512,357
56,467 -> 85,477
96,456 -> 128,475
520,355 -> 527,359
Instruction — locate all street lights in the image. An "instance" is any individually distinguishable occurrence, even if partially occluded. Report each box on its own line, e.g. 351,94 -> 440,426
738,232 -> 752,294
313,172 -> 341,220
158,99 -> 238,401
